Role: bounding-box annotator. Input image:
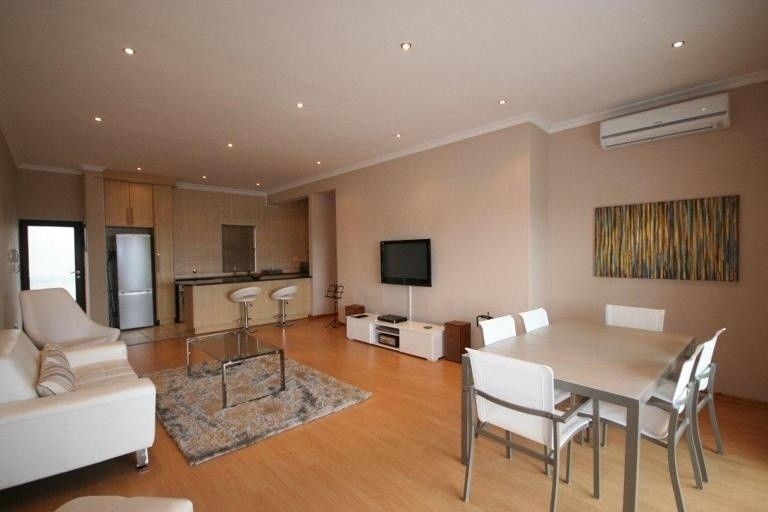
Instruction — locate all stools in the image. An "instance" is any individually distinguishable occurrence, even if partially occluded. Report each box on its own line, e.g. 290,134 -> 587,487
52,495 -> 194,512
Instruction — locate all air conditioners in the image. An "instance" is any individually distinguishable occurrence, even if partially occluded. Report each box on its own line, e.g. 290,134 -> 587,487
598,89 -> 736,152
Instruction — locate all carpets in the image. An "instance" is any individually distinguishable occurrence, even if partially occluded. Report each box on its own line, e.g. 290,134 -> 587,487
132,348 -> 375,468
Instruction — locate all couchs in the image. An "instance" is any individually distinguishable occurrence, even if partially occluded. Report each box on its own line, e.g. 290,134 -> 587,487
0,322 -> 158,494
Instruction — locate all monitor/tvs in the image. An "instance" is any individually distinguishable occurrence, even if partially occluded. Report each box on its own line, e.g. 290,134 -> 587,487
380,238 -> 431,287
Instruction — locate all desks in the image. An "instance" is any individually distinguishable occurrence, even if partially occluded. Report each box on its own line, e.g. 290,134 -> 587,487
462,316 -> 695,510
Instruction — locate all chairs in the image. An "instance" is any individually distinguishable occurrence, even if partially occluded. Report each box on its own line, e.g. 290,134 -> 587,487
518,307 -> 549,333
478,314 -> 516,345
461,349 -> 593,511
577,329 -> 728,512
270,285 -> 299,328
605,305 -> 666,333
17,285 -> 121,350
228,286 -> 265,335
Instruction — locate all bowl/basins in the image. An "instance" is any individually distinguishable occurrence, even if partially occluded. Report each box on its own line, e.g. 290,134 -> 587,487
249,273 -> 264,279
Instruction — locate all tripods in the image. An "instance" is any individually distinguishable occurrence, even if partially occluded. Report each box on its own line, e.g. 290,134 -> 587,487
325,298 -> 345,334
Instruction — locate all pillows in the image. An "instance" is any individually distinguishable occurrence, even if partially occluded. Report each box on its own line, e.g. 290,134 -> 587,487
39,342 -> 69,367
38,358 -> 75,398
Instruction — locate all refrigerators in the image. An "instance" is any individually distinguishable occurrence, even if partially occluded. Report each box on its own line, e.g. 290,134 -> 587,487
116,233 -> 154,331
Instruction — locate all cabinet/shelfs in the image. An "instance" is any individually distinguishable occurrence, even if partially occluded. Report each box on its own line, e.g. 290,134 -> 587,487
344,303 -> 365,316
103,178 -> 154,229
151,184 -> 177,326
444,320 -> 472,364
345,312 -> 446,364
